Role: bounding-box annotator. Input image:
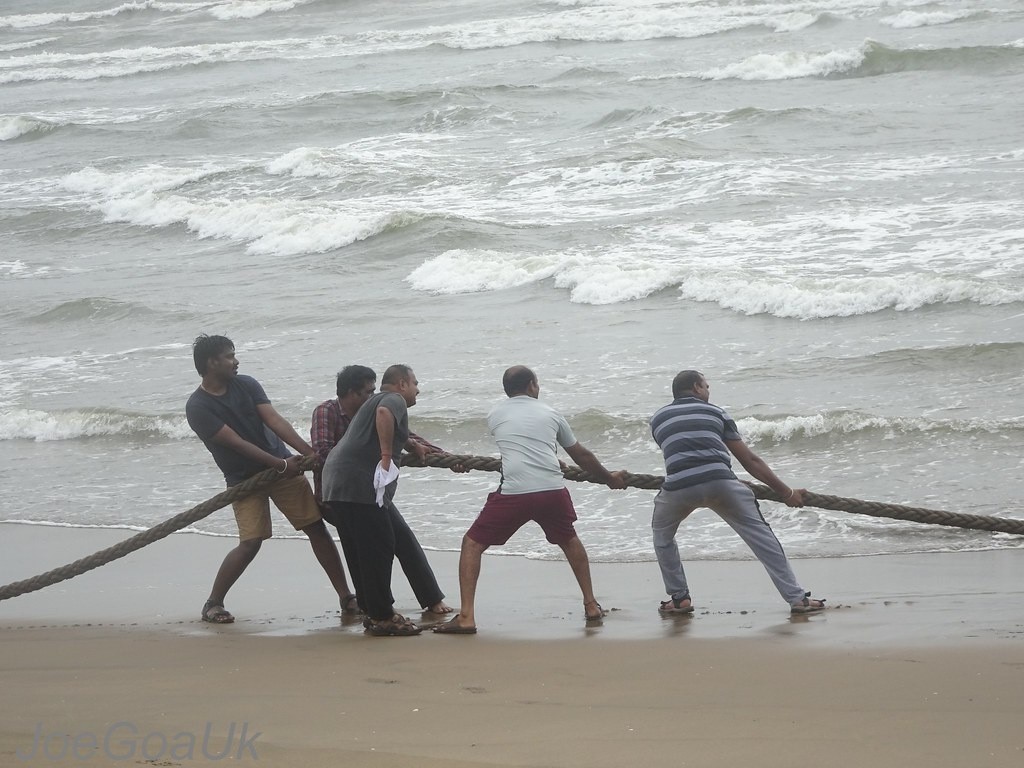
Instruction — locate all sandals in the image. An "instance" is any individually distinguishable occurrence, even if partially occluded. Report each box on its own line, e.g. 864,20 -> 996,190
660,594 -> 695,612
341,594 -> 366,615
201,601 -> 236,624
791,595 -> 827,612
364,614 -> 422,637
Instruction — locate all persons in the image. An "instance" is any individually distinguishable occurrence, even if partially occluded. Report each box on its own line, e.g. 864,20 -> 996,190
184,333 -> 825,638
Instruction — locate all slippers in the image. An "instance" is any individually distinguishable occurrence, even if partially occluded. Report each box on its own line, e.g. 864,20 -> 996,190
584,603 -> 603,620
433,615 -> 477,635
